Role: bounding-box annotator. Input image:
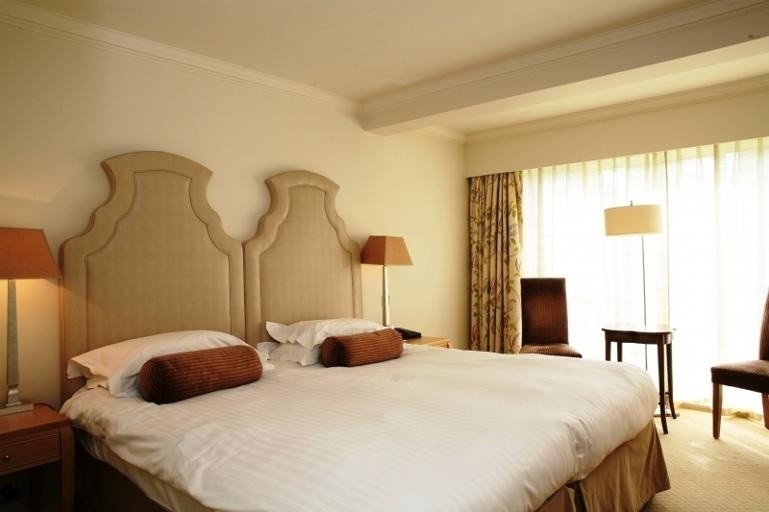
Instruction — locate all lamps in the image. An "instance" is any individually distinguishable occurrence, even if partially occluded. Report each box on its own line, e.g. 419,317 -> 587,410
604,204 -> 665,370
360,235 -> 414,326
0,226 -> 64,417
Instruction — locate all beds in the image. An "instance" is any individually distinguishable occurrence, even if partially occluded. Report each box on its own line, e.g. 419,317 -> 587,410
58,152 -> 671,512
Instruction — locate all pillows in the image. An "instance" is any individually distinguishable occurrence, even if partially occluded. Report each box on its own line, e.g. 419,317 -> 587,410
265,317 -> 388,351
257,341 -> 321,367
319,329 -> 404,367
66,330 -> 274,398
138,345 -> 262,404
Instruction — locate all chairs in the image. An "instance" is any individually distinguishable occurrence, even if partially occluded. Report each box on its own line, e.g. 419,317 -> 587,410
711,294 -> 769,439
518,277 -> 582,357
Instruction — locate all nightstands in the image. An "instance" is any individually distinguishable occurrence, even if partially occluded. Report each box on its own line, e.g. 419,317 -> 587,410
0,403 -> 76,512
405,336 -> 453,347
